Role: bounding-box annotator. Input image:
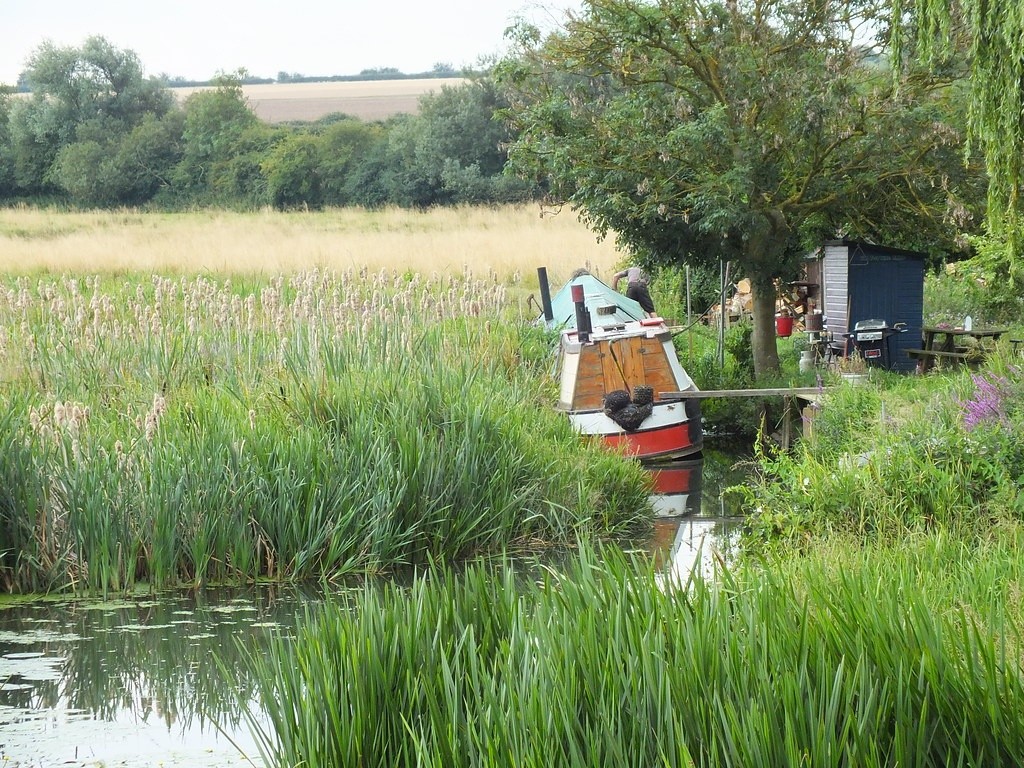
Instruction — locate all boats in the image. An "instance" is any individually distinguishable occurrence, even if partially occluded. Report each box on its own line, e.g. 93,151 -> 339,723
528,261 -> 706,462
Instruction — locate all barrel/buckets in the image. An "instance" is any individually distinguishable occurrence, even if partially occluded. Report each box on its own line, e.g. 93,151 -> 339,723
777,315 -> 794,338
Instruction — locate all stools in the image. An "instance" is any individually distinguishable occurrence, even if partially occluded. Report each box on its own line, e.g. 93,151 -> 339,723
1010,339 -> 1022,356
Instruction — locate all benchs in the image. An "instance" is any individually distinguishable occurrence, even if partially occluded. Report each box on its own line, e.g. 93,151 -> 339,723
903,348 -> 970,376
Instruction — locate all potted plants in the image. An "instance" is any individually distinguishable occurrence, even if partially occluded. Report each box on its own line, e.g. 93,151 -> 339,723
841,345 -> 870,387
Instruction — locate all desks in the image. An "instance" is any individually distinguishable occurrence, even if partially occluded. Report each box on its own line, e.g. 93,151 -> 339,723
919,327 -> 1009,353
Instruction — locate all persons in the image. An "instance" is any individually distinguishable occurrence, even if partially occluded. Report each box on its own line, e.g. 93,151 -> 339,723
611,266 -> 660,319
